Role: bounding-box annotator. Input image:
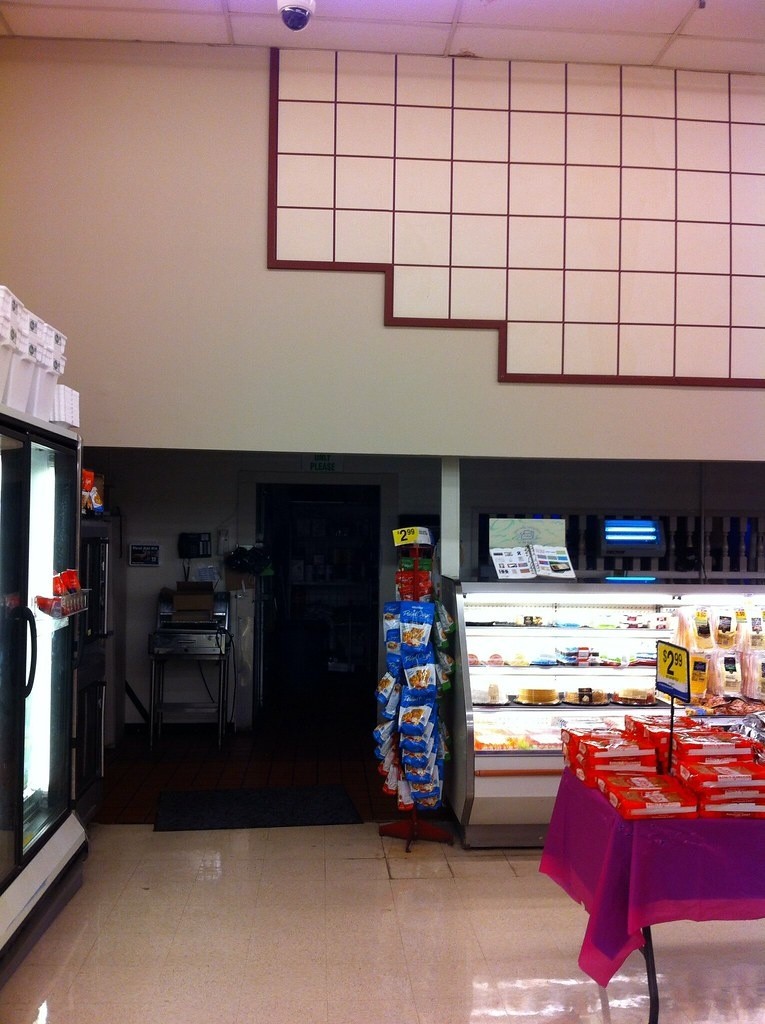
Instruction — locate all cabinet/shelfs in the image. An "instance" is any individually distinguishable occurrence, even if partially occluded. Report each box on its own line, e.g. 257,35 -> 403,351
443,575 -> 765,853
148,586 -> 231,751
284,532 -> 368,627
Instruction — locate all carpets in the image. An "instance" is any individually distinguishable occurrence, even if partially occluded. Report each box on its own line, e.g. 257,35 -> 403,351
155,785 -> 363,831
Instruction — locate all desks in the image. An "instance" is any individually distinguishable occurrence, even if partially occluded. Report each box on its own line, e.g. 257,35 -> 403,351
539,770 -> 765,1023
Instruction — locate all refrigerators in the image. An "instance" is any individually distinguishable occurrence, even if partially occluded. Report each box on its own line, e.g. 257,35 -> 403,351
0,403 -> 91,988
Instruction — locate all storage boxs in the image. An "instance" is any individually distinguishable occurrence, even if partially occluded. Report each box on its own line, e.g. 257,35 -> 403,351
561,714 -> 764,821
173,580 -> 214,609
0,285 -> 82,432
